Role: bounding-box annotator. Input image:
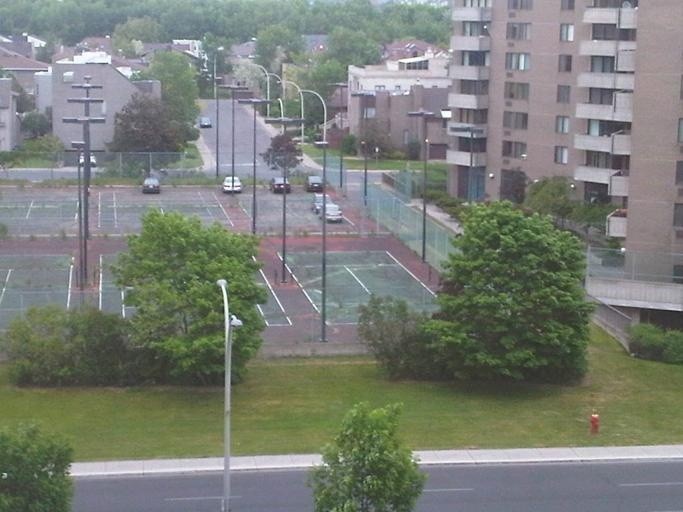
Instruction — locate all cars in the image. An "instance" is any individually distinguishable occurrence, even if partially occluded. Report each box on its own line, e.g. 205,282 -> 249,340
319,203 -> 343,222
142,175 -> 162,195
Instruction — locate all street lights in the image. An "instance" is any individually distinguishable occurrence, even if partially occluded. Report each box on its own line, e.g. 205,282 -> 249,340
449,125 -> 485,206
400,106 -> 436,267
475,171 -> 495,201
210,272 -> 247,512
358,139 -> 368,208
60,74 -> 109,290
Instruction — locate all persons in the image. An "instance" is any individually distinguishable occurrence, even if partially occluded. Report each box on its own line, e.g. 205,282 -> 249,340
591,409 -> 599,434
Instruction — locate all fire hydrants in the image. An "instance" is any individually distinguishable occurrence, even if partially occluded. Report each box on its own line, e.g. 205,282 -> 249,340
588,408 -> 601,433
